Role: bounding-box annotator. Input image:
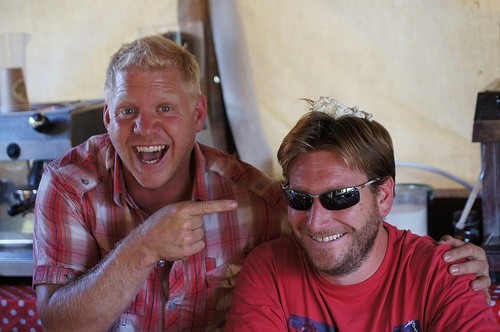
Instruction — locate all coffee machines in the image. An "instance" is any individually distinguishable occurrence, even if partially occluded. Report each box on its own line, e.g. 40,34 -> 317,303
0,100 -> 106,287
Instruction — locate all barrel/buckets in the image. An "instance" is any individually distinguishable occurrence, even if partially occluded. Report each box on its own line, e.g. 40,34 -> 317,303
378,183 -> 428,242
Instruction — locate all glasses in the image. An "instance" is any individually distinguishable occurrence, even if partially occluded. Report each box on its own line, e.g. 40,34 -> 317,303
281,177 -> 382,212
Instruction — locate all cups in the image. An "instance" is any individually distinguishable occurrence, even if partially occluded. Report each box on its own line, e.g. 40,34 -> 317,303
3,66 -> 30,113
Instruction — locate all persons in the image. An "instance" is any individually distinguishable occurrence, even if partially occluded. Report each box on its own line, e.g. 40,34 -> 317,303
31,35 -> 491,332
226,112 -> 500,332
